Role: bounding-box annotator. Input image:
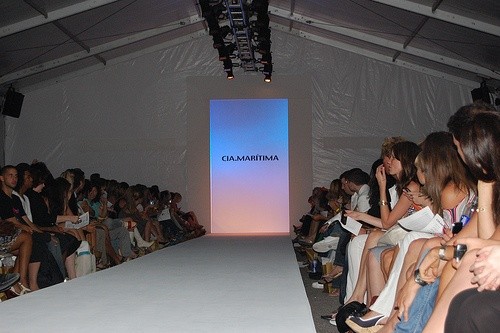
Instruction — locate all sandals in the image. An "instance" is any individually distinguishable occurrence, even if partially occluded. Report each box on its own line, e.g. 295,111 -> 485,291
345,316 -> 385,333
10,282 -> 31,295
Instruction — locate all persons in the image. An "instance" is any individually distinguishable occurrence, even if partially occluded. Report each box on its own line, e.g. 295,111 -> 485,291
291,103 -> 500,333
0,159 -> 207,296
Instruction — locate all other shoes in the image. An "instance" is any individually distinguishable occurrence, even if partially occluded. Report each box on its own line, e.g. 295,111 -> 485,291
299,239 -> 313,245
322,269 -> 343,277
157,239 -> 169,245
125,252 -> 139,258
312,236 -> 339,252
328,289 -> 340,295
138,241 -> 155,248
312,281 -> 324,288
320,307 -> 336,319
123,221 -> 137,228
330,318 -> 336,326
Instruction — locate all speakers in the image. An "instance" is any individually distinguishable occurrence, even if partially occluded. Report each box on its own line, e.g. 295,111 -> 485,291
471,87 -> 491,105
2,89 -> 25,118
